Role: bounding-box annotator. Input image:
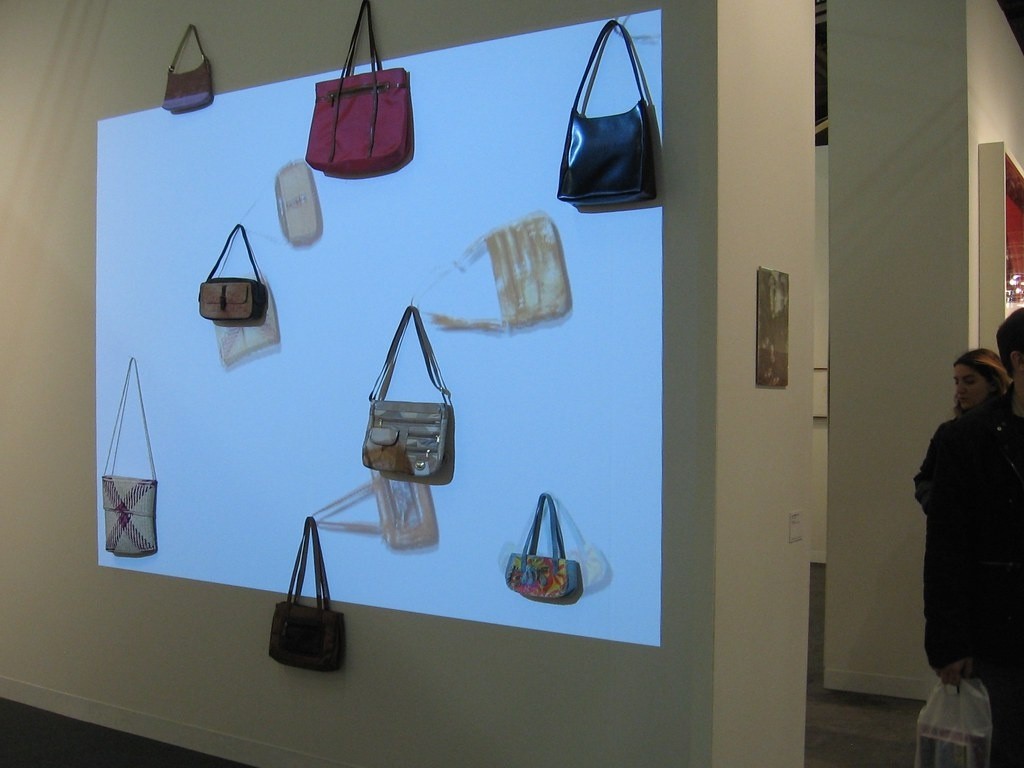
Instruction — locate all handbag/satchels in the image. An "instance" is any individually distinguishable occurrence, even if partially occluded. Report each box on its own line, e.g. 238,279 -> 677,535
101,357 -> 157,554
268,515 -> 346,672
361,307 -> 452,479
162,25 -> 213,111
236,160 -> 322,248
411,208 -> 572,331
556,17 -> 655,209
504,493 -> 577,600
198,224 -> 266,322
306,0 -> 415,180
214,228 -> 280,367
311,469 -> 440,549
914,665 -> 992,768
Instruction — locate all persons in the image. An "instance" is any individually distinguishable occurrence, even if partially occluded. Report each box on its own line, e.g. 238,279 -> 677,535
914,347 -> 1013,522
922,307 -> 1024,768
